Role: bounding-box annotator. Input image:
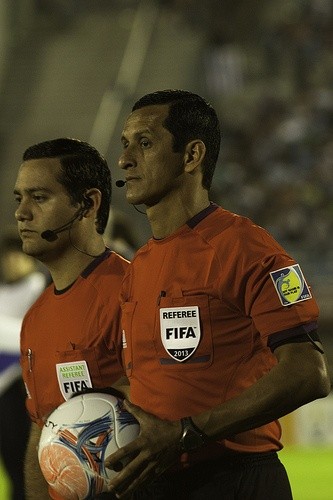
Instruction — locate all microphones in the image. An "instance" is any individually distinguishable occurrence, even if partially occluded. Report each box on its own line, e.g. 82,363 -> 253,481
115,180 -> 127,187
41,204 -> 88,239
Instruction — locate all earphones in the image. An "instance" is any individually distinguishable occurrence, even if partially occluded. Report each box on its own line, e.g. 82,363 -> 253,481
84,198 -> 94,208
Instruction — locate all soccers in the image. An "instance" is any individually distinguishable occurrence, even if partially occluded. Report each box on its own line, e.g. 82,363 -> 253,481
38,393 -> 141,500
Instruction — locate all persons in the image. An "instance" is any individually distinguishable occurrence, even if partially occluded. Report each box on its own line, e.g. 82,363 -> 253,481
98,88 -> 333,500
0,232 -> 46,500
14,134 -> 134,500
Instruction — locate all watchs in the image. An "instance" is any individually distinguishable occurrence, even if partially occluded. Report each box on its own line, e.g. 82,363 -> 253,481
179,417 -> 209,456
